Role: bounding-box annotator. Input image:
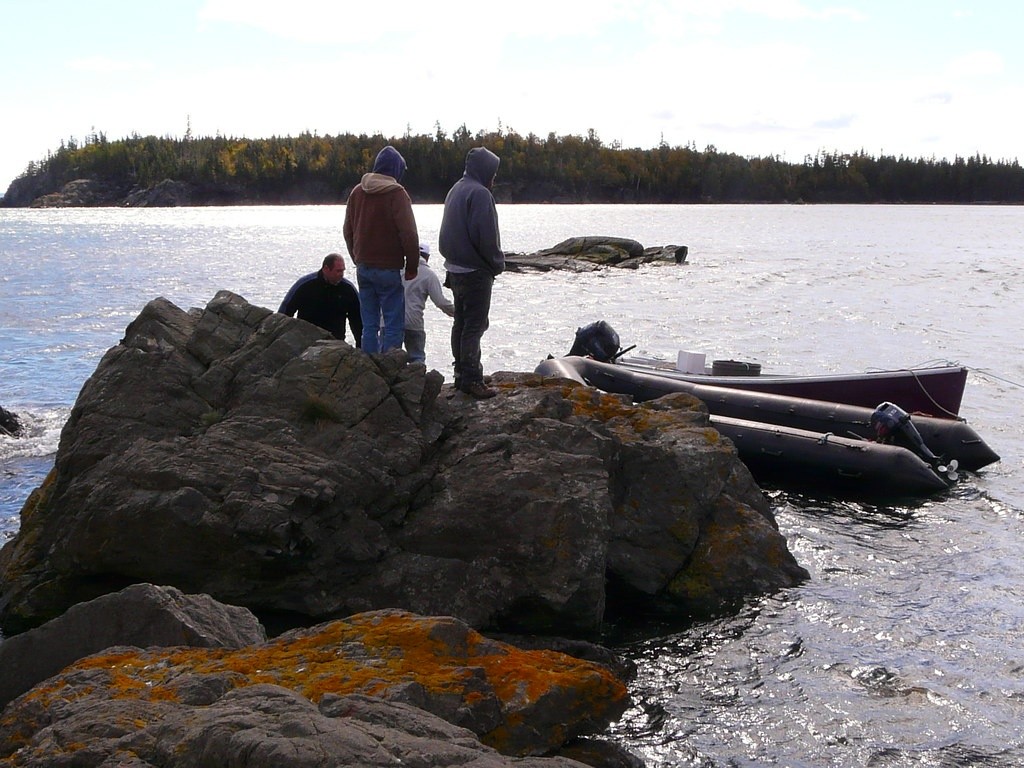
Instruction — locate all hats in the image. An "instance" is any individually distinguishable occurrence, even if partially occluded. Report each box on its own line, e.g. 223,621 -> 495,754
419,243 -> 430,253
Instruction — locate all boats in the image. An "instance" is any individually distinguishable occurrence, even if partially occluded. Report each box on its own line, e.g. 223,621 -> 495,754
571,321 -> 968,419
536,355 -> 1002,495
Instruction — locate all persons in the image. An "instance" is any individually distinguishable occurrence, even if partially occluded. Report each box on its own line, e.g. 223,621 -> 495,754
343,146 -> 419,354
278,253 -> 363,348
380,244 -> 455,364
439,147 -> 505,399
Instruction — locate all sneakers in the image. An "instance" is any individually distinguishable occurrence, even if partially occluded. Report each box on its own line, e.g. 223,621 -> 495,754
455,375 -> 496,399
